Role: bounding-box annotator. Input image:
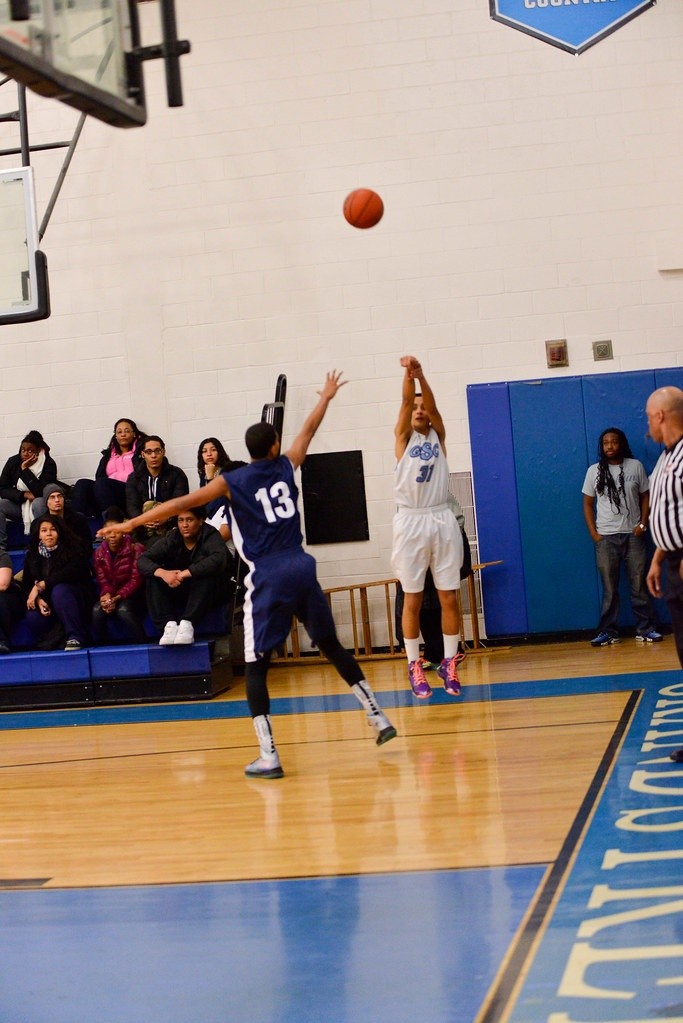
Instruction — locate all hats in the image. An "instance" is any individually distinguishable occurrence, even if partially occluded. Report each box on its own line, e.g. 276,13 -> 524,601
44,483 -> 64,504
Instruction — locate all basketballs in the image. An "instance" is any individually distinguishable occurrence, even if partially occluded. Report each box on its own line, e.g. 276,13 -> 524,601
343,188 -> 384,229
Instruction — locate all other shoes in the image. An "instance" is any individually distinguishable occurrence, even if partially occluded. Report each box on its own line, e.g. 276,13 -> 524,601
670,749 -> 683,764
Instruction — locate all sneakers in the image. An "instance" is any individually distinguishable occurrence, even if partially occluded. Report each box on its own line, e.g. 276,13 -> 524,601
590,632 -> 622,647
159,624 -> 194,645
376,721 -> 397,747
408,658 -> 433,699
0,642 -> 9,655
636,628 -> 663,642
65,639 -> 81,651
244,755 -> 283,779
437,654 -> 466,696
419,646 -> 467,670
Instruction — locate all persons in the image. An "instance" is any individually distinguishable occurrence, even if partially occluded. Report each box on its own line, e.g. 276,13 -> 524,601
390,355 -> 466,698
97,368 -> 397,779
0,419 -> 248,653
644,385 -> 683,762
581,428 -> 664,646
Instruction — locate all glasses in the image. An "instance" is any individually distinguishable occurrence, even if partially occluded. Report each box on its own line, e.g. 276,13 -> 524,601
143,448 -> 163,454
116,429 -> 133,434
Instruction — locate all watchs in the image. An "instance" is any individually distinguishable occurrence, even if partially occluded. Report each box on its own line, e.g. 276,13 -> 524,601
640,524 -> 648,532
